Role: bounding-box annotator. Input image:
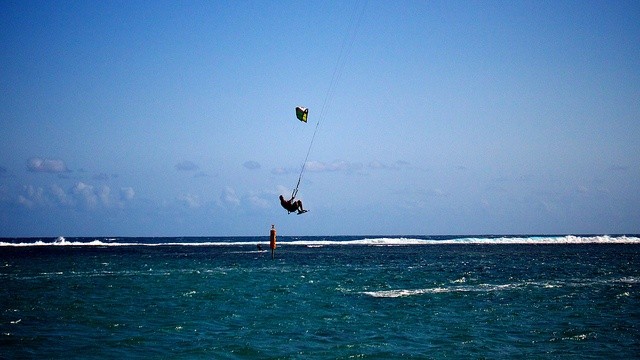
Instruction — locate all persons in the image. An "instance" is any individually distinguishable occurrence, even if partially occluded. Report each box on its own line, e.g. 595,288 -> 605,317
279,194 -> 308,214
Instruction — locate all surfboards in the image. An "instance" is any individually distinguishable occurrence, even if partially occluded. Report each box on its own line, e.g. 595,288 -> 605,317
297,210 -> 308,215
